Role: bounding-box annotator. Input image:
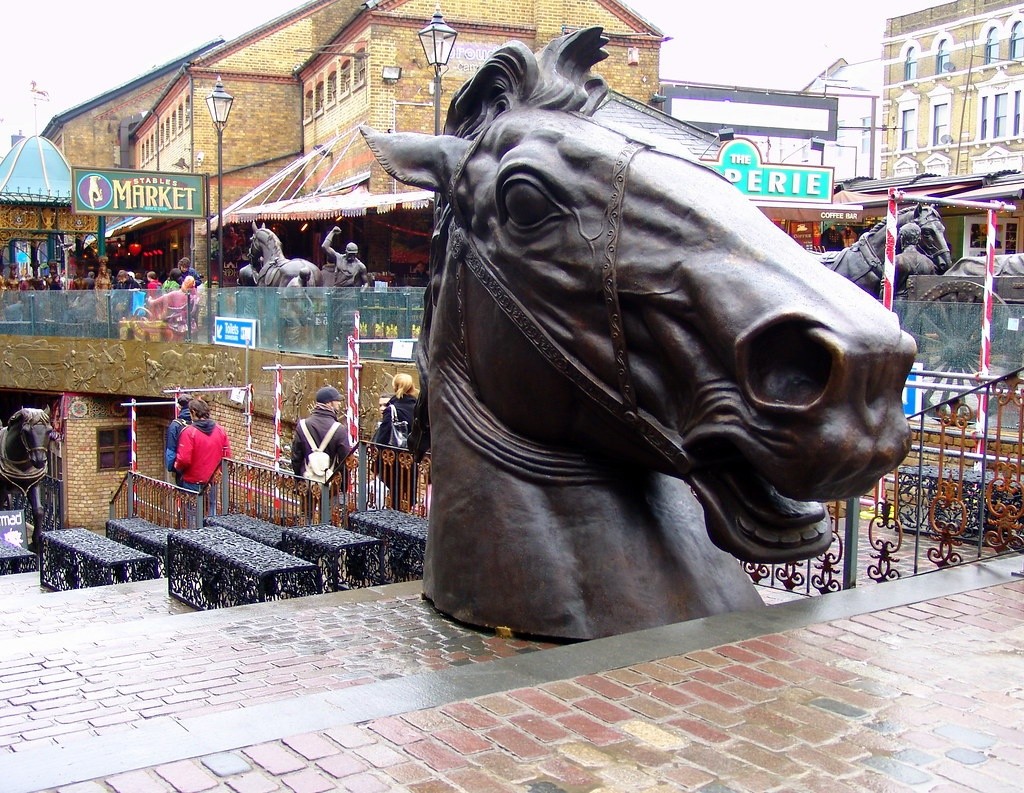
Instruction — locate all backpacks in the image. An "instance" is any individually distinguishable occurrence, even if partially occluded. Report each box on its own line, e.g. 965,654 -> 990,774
299,418 -> 342,492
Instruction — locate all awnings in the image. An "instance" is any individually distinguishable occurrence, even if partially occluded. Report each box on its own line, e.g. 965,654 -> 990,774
831,181 -> 1024,218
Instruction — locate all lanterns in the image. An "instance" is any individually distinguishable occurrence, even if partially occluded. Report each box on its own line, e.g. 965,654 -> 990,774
83,243 -> 163,259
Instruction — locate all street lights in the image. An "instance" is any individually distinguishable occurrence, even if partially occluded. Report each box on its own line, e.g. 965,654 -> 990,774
420,5 -> 460,229
206,74 -> 235,286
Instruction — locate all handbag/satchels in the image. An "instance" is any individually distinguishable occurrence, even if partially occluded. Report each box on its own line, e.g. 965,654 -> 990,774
378,404 -> 409,448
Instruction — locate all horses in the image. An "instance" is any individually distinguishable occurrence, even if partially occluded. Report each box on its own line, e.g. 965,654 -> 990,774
356,24 -> 919,643
802,200 -> 953,298
246,219 -> 325,295
0,403 -> 55,556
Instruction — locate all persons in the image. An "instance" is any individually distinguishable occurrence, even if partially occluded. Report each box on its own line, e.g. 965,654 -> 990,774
283,268 -> 316,353
376,373 -> 418,506
880,223 -> 935,369
291,386 -> 349,526
315,253 -> 341,342
165,394 -> 232,528
0,258 -> 202,341
148,276 -> 200,342
411,262 -> 431,287
321,226 -> 367,354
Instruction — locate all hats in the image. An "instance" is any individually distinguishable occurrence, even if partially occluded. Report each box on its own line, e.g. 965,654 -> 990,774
316,387 -> 345,403
177,394 -> 193,408
